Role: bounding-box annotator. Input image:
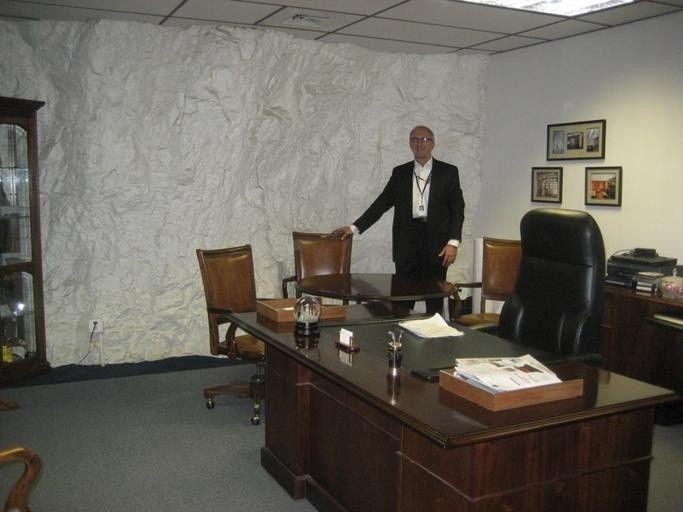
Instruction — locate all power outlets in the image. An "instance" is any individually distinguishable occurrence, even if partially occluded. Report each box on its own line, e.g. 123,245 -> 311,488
88,318 -> 103,335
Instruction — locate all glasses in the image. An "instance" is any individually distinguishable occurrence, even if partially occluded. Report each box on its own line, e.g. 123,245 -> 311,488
409,138 -> 433,143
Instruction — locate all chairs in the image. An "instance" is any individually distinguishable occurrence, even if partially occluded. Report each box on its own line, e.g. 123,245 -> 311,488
450,237 -> 524,331
483,209 -> 607,363
0,444 -> 41,512
282,231 -> 352,307
196,245 -> 267,425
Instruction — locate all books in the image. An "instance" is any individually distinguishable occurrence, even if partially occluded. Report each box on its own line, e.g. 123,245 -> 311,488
636,271 -> 665,292
454,353 -> 563,394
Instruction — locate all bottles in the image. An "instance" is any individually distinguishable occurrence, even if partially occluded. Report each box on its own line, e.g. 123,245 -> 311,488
388,342 -> 402,368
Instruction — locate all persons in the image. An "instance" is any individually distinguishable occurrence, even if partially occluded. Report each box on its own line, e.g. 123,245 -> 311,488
332,125 -> 465,316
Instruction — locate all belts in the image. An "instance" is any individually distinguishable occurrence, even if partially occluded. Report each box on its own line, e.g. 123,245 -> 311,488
411,218 -> 428,224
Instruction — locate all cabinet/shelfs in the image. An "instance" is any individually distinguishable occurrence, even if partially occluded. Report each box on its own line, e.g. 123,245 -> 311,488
0,95 -> 50,387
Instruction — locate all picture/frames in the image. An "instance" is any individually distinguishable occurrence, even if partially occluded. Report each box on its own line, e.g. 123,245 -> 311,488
531,167 -> 562,203
546,119 -> 606,161
585,167 -> 622,207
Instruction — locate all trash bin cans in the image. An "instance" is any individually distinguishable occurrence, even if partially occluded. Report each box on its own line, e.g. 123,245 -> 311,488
448,296 -> 472,319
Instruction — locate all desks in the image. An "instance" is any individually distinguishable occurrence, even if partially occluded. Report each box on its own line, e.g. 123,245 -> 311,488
599,277 -> 682,427
223,301 -> 682,512
294,274 -> 458,307
